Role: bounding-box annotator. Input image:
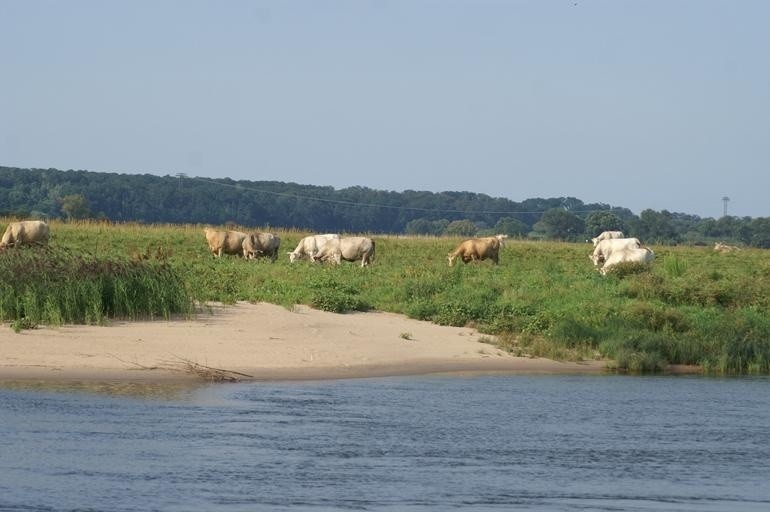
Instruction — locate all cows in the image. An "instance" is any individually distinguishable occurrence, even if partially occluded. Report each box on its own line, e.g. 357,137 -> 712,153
242,233 -> 280,264
0,220 -> 49,250
286,234 -> 339,264
714,242 -> 741,253
588,231 -> 655,275
204,227 -> 246,259
446,237 -> 499,267
497,234 -> 508,249
312,237 -> 375,274
